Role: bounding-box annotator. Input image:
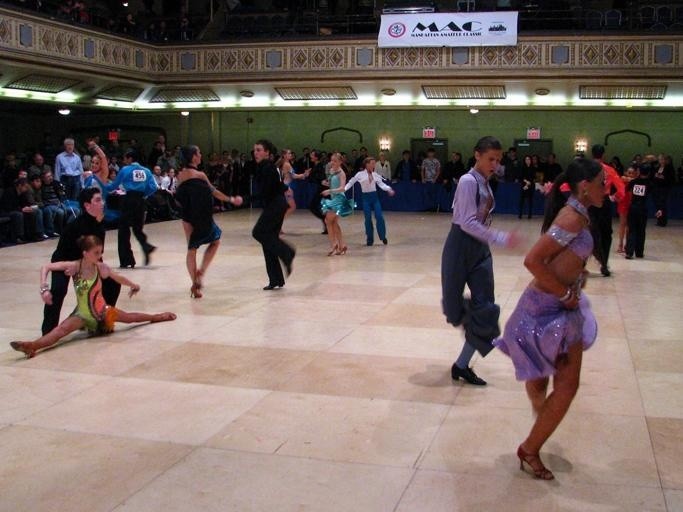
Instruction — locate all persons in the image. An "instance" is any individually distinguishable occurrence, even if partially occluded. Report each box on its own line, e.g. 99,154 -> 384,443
305,150 -> 331,234
466,147 -> 476,171
443,153 -> 455,212
26,174 -> 60,239
320,152 -> 354,257
492,158 -> 607,481
530,155 -> 543,175
631,154 -> 642,165
0,178 -> 44,244
455,153 -> 464,190
173,144 -> 241,298
275,149 -> 312,235
81,154 -> 92,171
345,157 -> 395,246
649,153 -> 675,227
62,140 -> 122,225
421,148 -> 441,184
518,154 -> 536,220
128,136 -> 181,165
27,154 -> 51,179
200,148 -> 257,210
10,235 -> 177,359
291,147 -> 370,170
500,147 -> 518,183
624,164 -> 661,259
42,187 -> 121,338
394,151 -> 417,185
104,151 -> 159,270
614,164 -> 637,253
3,158 -> 18,177
109,156 -> 120,171
543,153 -> 562,213
151,165 -> 179,220
440,135 -> 503,386
574,151 -> 585,160
50,172 -> 63,200
374,151 -> 391,181
54,139 -> 86,201
242,139 -> 296,291
584,145 -> 626,277
107,166 -> 126,195
610,156 -> 624,176
161,167 -> 177,214
40,171 -> 65,211
642,155 -> 661,177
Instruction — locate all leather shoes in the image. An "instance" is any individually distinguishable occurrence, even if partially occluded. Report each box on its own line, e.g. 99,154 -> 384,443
144,246 -> 156,265
285,249 -> 296,275
263,282 -> 284,290
120,263 -> 135,267
451,362 -> 487,386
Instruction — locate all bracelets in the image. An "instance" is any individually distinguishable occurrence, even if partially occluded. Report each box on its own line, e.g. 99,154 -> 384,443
559,290 -> 570,302
230,196 -> 235,204
39,287 -> 48,294
40,283 -> 48,287
564,294 -> 574,304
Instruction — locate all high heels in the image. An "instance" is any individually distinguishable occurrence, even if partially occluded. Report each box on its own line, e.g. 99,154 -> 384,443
190,285 -> 202,298
335,246 -> 348,256
327,244 -> 338,256
9,341 -> 36,358
151,313 -> 177,321
517,445 -> 554,481
194,270 -> 203,290
